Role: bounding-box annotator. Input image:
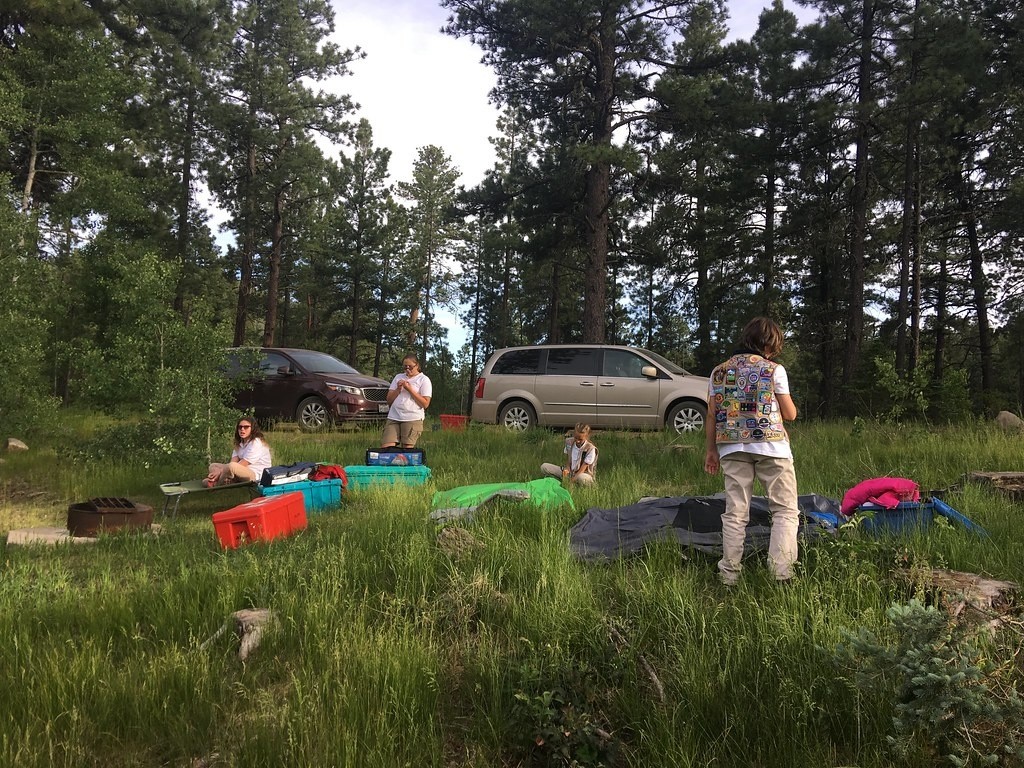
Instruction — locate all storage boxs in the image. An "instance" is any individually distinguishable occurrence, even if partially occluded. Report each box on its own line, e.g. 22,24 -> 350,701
211,491 -> 308,550
858,497 -> 986,538
365,447 -> 426,465
258,478 -> 341,515
440,414 -> 470,430
344,465 -> 433,493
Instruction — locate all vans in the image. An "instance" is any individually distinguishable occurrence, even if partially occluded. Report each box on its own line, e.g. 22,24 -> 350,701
469,343 -> 713,439
211,349 -> 394,433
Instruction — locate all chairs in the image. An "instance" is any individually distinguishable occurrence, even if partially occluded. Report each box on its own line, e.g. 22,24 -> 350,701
612,360 -> 648,377
159,475 -> 254,518
260,362 -> 333,375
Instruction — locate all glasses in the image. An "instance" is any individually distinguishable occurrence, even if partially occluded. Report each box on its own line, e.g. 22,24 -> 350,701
238,425 -> 251,429
402,364 -> 417,370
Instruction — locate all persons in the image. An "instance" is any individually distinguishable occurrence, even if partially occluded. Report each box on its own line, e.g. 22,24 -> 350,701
540,422 -> 599,486
705,317 -> 801,592
381,353 -> 432,448
202,416 -> 272,488
